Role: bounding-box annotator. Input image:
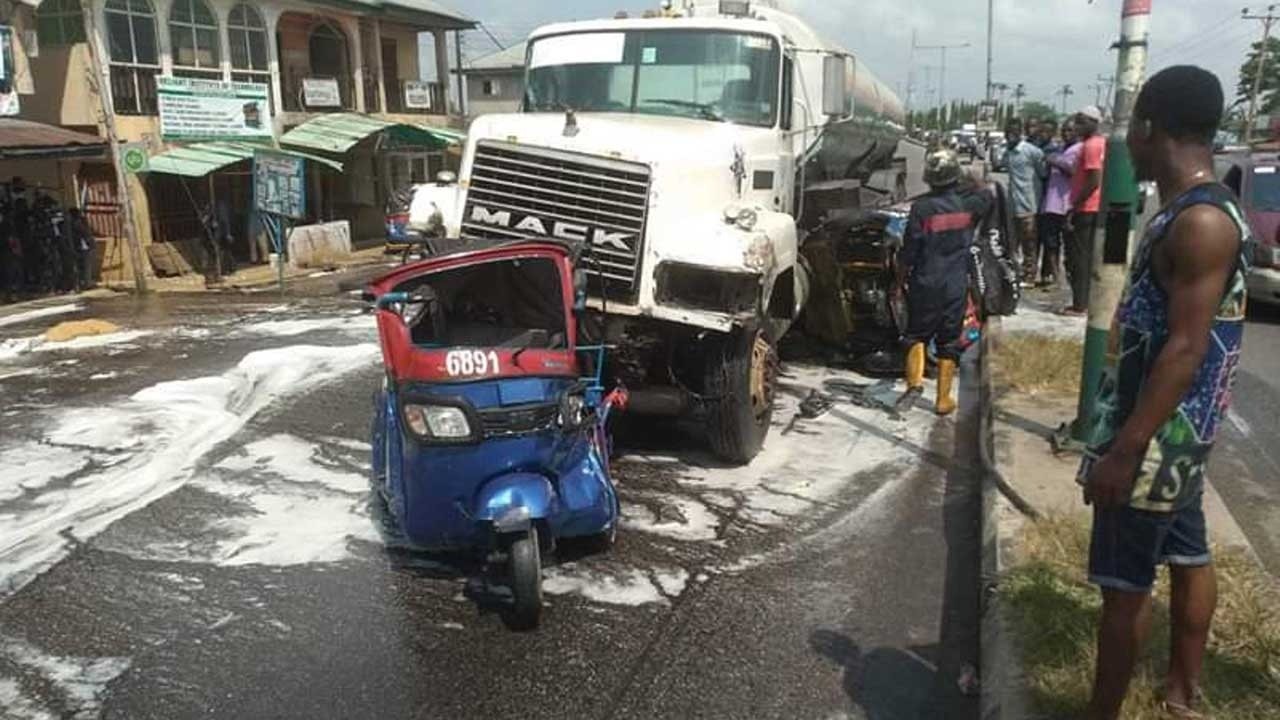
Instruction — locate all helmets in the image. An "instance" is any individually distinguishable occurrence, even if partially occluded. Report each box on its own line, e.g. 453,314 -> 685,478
923,151 -> 961,186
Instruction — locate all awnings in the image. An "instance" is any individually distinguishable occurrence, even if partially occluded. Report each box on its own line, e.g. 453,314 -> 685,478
280,111 -> 466,153
145,141 -> 344,176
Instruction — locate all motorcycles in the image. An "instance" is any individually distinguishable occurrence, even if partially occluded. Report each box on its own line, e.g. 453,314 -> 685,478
369,235 -> 632,632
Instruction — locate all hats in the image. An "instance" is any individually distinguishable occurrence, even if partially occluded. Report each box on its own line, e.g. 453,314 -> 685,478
1080,106 -> 1102,122
437,169 -> 456,183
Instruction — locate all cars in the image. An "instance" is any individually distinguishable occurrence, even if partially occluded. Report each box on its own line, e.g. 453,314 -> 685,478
1211,149 -> 1280,309
915,122 -> 1005,153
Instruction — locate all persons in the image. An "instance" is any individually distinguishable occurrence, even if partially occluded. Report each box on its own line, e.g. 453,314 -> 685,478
894,148 -> 993,414
950,104 -> 1105,318
69,207 -> 97,290
1075,66 -> 1253,720
203,184 -> 269,272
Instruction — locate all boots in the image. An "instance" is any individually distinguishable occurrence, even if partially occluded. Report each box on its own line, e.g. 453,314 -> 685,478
896,341 -> 925,411
935,358 -> 955,413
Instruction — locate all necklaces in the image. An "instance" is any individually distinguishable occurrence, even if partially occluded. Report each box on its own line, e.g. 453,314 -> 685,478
1160,169 -> 1215,203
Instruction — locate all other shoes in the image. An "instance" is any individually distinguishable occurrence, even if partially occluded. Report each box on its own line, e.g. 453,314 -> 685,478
1018,278 -> 1037,288
1058,306 -> 1086,318
1042,275 -> 1057,291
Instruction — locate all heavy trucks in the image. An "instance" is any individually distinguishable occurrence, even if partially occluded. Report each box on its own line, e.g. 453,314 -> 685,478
382,0 -> 934,469
